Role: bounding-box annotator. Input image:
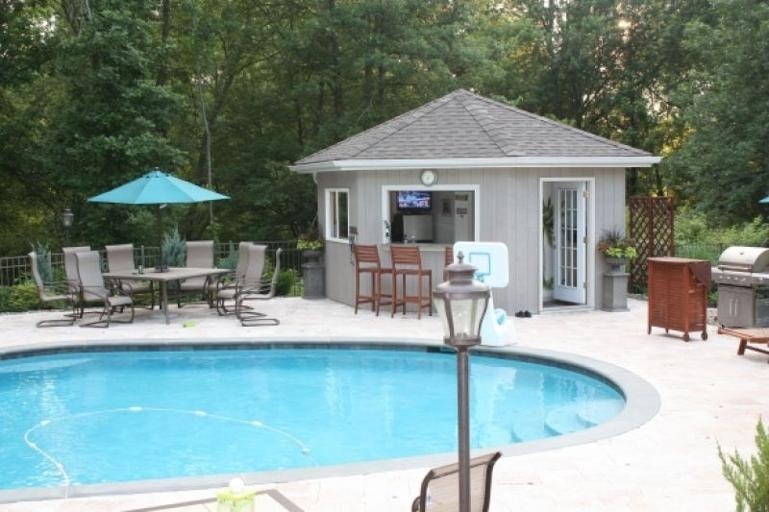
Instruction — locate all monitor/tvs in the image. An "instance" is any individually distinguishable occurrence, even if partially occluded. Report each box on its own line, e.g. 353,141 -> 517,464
396,191 -> 432,211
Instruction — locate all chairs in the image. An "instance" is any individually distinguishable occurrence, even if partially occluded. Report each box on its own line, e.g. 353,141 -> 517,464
717,324 -> 769,355
351,240 -> 396,317
412,452 -> 501,512
444,246 -> 453,281
390,244 -> 432,320
28,240 -> 282,327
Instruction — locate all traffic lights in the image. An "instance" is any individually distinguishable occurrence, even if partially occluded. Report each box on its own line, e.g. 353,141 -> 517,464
62,208 -> 75,245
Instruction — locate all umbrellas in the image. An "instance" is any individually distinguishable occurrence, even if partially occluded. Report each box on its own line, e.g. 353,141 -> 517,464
87,167 -> 232,309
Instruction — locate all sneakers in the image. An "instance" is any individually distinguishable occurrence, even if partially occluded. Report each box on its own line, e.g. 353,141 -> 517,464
515,310 -> 531,317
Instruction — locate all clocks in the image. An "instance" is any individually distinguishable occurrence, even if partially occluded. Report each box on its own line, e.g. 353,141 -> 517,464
421,170 -> 437,185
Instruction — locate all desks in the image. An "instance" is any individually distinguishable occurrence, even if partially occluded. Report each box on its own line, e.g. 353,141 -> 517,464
647,257 -> 711,342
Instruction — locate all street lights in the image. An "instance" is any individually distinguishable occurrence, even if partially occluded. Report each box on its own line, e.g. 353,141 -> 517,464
431,249 -> 491,511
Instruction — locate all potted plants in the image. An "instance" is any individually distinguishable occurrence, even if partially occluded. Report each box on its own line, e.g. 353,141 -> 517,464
596,225 -> 637,272
297,233 -> 324,265
